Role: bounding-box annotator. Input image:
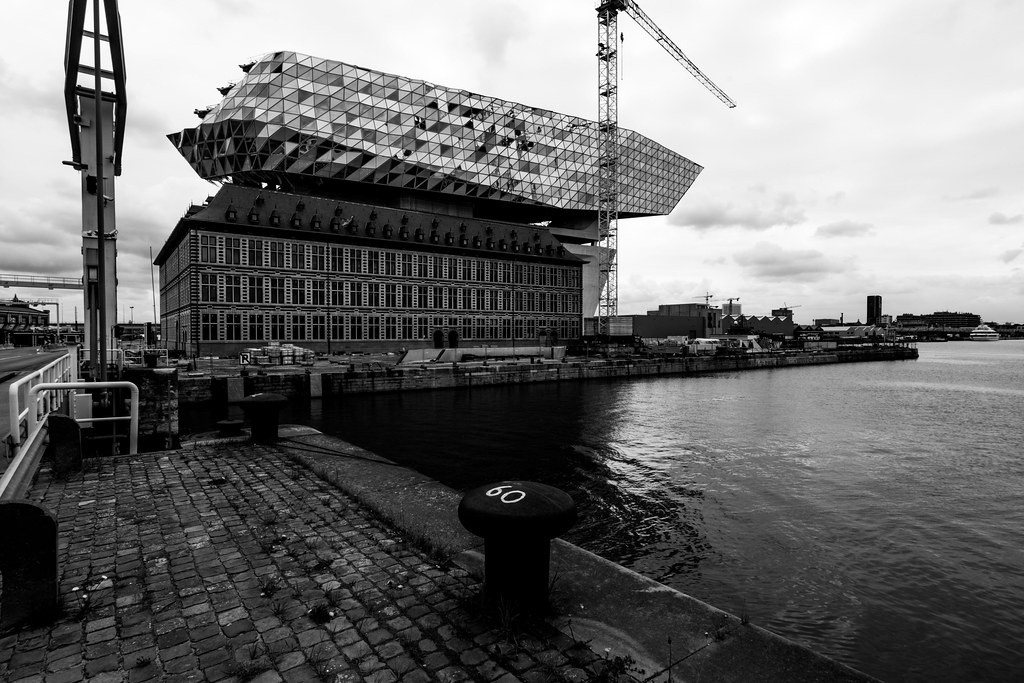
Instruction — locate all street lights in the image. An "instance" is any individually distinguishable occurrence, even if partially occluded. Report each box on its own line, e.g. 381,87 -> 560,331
130,307 -> 134,339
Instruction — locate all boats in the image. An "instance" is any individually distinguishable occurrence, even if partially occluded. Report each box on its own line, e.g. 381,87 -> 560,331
969,324 -> 999,341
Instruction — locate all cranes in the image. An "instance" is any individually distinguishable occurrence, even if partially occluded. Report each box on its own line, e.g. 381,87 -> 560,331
694,292 -> 713,304
596,0 -> 736,316
715,297 -> 740,316
